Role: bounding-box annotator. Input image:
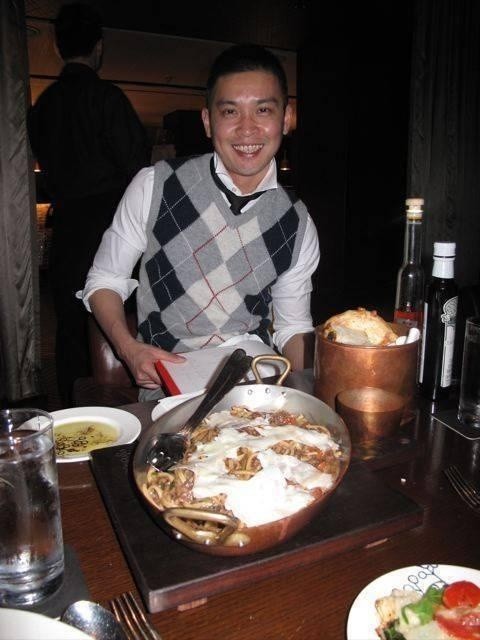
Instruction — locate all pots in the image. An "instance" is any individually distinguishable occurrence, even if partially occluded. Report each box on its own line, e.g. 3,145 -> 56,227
133,355 -> 353,558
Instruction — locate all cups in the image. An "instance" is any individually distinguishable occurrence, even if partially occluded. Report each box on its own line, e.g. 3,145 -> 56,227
336,385 -> 406,463
456,318 -> 480,430
0,409 -> 64,608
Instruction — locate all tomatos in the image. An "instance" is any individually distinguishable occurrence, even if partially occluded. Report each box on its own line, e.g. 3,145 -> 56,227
442,580 -> 480,609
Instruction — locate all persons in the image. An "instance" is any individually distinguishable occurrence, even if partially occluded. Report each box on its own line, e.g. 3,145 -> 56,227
26,4 -> 152,409
72,43 -> 319,404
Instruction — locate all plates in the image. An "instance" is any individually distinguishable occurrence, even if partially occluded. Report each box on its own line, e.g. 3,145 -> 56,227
346,563 -> 479,639
18,406 -> 142,465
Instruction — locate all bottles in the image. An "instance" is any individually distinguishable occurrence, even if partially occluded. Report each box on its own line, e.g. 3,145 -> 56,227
395,198 -> 464,402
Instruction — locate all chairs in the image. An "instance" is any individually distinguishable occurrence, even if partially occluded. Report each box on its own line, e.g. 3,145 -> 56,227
82,309 -> 139,387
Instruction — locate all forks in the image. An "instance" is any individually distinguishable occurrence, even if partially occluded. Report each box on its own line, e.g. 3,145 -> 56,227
111,591 -> 161,639
445,465 -> 479,511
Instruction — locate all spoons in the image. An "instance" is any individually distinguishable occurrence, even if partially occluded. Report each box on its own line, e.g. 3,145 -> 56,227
62,600 -> 130,640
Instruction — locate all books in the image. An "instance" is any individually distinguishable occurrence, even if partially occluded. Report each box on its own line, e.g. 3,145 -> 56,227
156,342 -> 288,396
